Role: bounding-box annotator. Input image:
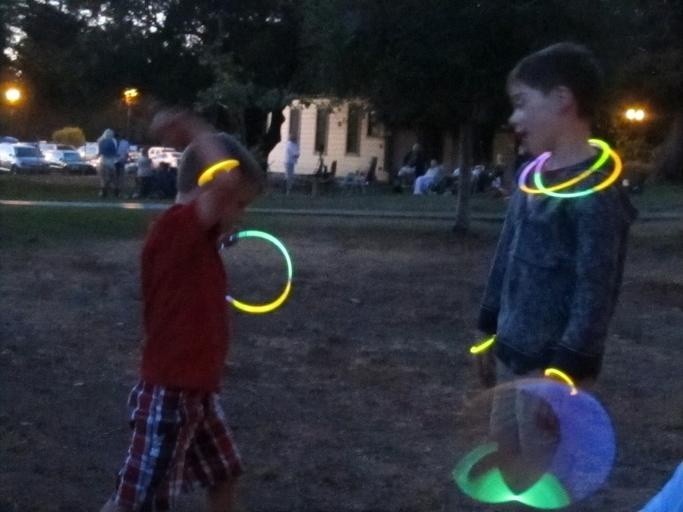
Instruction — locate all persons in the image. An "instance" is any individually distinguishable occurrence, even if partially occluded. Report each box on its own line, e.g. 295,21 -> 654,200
76,124 -> 177,200
97,101 -> 266,510
391,141 -> 508,195
468,36 -> 633,493
284,130 -> 299,195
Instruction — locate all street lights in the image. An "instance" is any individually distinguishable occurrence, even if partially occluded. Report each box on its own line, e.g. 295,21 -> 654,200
4,84 -> 20,136
120,87 -> 140,143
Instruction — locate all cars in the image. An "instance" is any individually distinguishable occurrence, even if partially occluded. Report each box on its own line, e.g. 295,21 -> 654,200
0,140 -> 179,176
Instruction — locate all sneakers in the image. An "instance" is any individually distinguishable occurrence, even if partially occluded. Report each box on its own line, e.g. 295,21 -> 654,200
97,188 -> 144,201
